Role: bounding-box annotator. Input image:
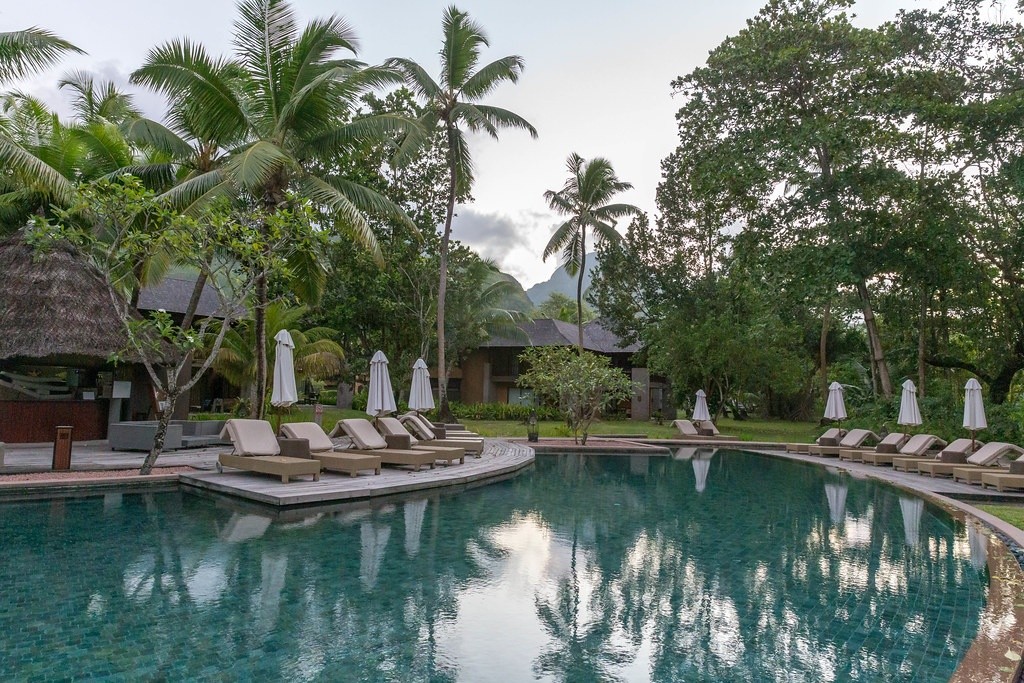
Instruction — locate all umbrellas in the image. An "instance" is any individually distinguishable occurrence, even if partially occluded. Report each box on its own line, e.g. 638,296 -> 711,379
962,378 -> 988,452
407,357 -> 436,418
899,497 -> 925,545
692,389 -> 710,428
823,381 -> 848,442
825,475 -> 849,523
365,350 -> 397,430
404,499 -> 428,561
271,329 -> 296,436
692,451 -> 711,492
897,379 -> 923,445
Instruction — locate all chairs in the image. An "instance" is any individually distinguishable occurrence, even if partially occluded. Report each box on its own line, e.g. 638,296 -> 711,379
692,420 -> 740,441
217,409 -> 485,483
786,428 -> 1024,492
669,419 -> 716,439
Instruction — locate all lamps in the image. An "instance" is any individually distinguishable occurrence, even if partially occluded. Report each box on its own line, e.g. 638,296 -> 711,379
528,408 -> 539,443
